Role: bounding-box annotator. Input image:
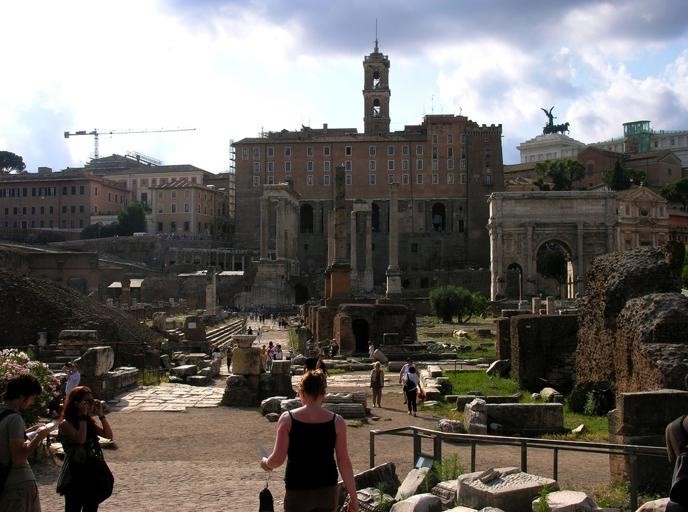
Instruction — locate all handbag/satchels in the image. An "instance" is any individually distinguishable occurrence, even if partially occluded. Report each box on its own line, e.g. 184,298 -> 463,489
405,380 -> 416,392
259,488 -> 274,512
72,457 -> 113,505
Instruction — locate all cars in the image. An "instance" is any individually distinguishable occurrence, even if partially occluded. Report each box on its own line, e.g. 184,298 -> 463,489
132,232 -> 149,236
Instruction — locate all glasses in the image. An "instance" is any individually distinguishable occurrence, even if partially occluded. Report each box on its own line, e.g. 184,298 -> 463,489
75,399 -> 95,405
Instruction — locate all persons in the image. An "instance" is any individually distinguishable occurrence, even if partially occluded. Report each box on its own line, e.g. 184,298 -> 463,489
61,361 -> 80,420
55,386 -> 113,511
202,293 -> 342,379
259,367 -> 361,512
665,411 -> 688,511
367,341 -> 375,358
370,362 -> 385,408
0,373 -> 50,511
403,367 -> 425,417
399,356 -> 420,404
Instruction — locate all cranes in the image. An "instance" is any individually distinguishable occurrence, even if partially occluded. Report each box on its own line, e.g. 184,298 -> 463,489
62,128 -> 199,161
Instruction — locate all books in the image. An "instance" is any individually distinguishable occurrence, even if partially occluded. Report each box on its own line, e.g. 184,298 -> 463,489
23,421 -> 57,440
